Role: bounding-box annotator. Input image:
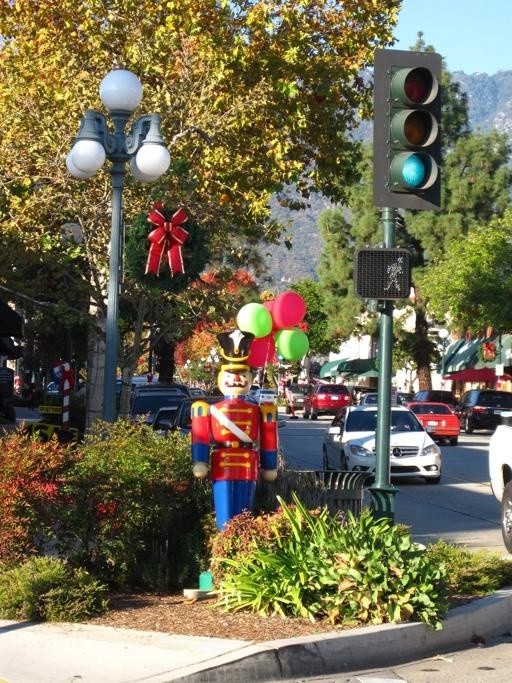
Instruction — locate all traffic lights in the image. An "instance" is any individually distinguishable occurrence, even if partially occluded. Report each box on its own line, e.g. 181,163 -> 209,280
372,49 -> 442,211
353,248 -> 412,301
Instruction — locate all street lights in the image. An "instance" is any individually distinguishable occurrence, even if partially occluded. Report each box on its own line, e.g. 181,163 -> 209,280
439,329 -> 449,377
66,69 -> 170,420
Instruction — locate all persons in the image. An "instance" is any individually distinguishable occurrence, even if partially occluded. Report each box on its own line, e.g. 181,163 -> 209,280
192,331 -> 278,567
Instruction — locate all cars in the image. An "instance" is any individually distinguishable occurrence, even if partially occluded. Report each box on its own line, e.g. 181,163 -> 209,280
46,381 -> 62,396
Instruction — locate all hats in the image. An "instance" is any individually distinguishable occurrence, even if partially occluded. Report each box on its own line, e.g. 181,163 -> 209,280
212,326 -> 254,372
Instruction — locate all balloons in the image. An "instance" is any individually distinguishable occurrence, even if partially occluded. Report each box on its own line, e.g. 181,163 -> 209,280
237,290 -> 309,366
